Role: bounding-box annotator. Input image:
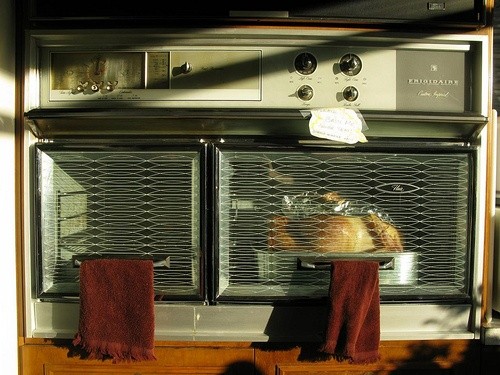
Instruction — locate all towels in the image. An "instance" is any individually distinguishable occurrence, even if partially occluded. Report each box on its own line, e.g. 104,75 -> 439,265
70,259 -> 156,364
319,260 -> 380,367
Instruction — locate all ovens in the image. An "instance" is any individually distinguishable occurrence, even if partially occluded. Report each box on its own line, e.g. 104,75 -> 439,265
15,20 -> 494,341
